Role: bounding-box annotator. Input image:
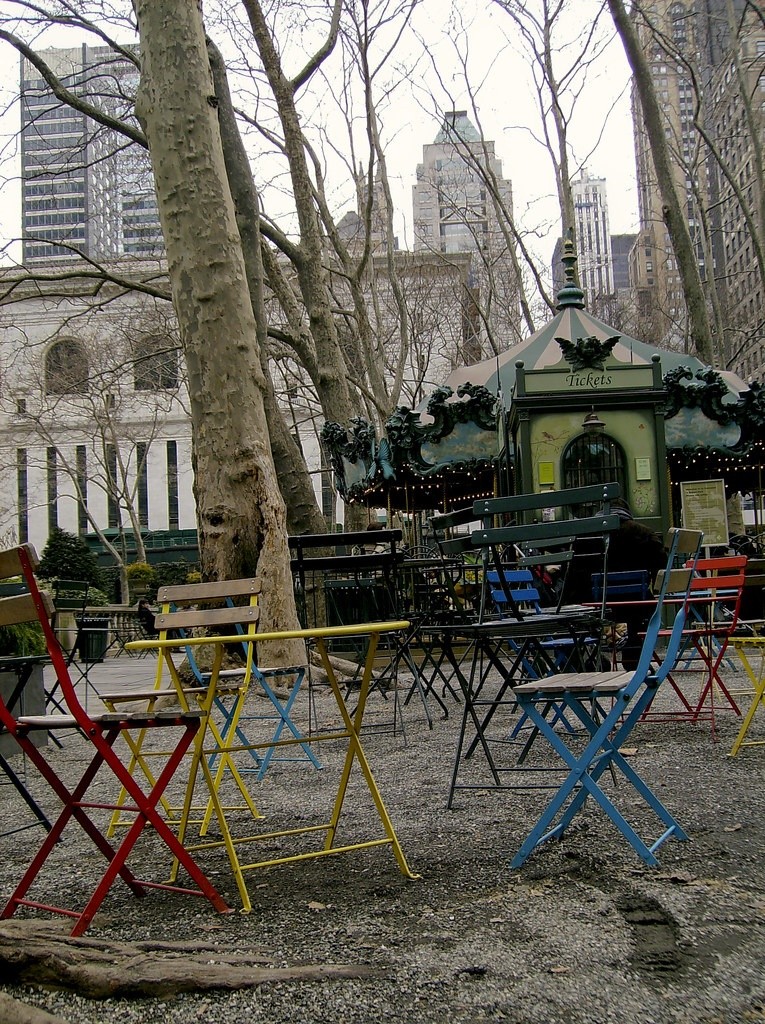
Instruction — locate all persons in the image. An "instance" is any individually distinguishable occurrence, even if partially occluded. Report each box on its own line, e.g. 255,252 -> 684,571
560,497 -> 668,676
349,522 -> 407,626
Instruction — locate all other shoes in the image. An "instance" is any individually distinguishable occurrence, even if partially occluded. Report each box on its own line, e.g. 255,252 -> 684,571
587,656 -> 611,673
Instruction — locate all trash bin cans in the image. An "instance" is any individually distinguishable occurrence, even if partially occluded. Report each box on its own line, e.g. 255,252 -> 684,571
75,616 -> 109,662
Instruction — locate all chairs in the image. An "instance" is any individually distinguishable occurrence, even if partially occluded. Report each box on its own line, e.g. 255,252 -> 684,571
0,480 -> 764,944
